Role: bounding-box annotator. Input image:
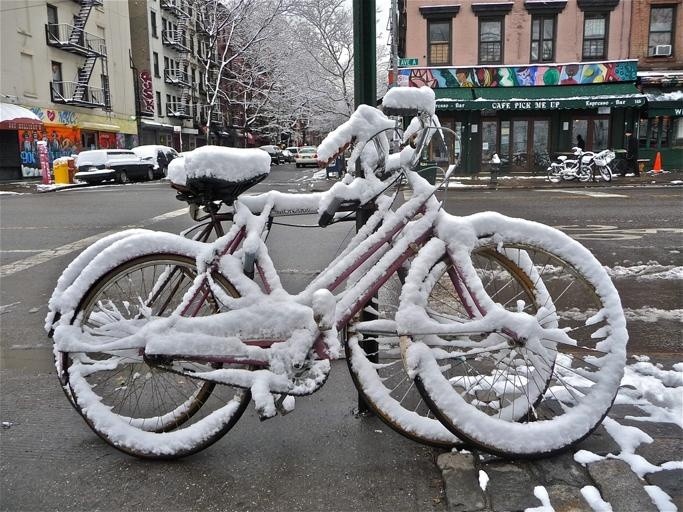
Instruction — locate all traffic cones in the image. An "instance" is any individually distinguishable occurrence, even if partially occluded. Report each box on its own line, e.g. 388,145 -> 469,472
650,152 -> 662,173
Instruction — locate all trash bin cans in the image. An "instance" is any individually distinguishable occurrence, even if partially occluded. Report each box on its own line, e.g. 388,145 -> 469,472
419,160 -> 436,185
326,151 -> 346,179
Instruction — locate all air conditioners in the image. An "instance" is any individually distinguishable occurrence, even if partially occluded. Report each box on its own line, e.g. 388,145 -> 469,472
653,45 -> 671,57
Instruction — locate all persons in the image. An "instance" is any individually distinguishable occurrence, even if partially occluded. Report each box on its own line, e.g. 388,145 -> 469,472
19,130 -> 59,151
576,135 -> 585,150
